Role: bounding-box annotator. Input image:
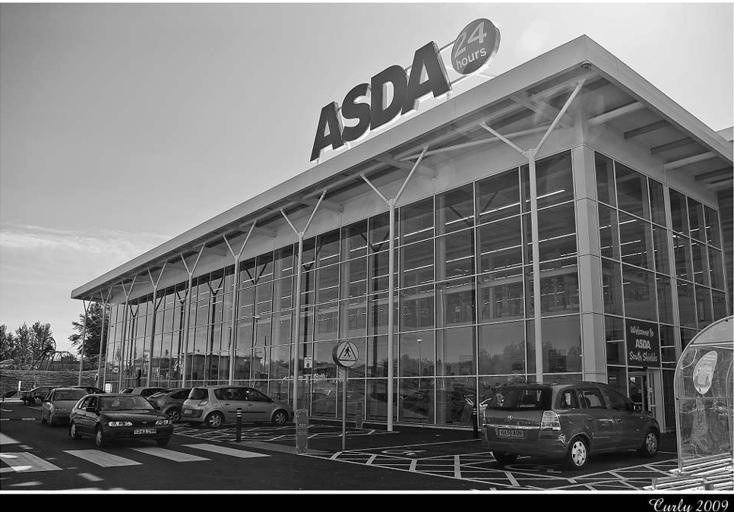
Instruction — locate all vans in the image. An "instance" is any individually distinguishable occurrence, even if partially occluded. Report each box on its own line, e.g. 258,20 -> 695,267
482,380 -> 662,470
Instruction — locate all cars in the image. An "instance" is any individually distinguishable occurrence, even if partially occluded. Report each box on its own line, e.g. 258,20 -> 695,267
19,382 -> 292,451
1,387 -> 25,398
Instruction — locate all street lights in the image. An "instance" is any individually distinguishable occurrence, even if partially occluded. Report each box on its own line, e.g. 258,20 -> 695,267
416,339 -> 423,390
